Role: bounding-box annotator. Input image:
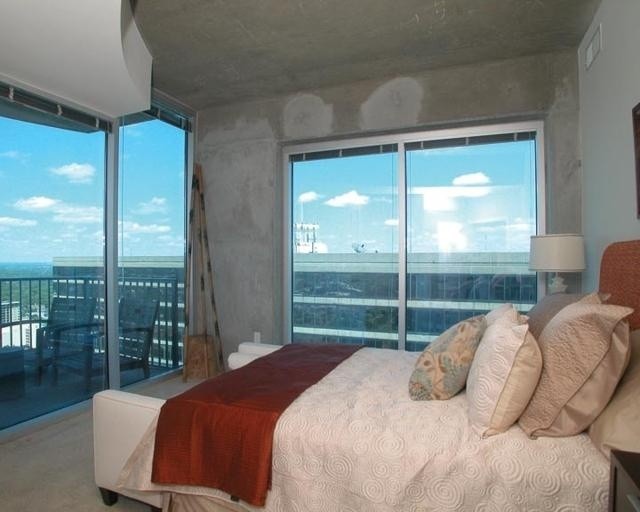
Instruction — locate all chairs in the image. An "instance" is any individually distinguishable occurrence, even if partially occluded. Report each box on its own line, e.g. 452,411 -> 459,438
51,295 -> 158,394
24,297 -> 97,387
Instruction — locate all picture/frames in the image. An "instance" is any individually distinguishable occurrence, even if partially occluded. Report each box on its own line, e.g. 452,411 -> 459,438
632,102 -> 640,219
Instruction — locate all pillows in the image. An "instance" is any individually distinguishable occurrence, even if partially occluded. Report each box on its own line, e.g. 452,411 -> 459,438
408,291 -> 635,441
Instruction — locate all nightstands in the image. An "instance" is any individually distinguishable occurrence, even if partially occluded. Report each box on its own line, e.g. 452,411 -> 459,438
607,449 -> 640,512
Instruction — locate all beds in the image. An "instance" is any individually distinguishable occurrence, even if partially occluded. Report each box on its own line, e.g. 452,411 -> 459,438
151,239 -> 640,512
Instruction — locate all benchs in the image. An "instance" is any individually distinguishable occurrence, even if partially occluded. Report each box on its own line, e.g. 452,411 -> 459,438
93,342 -> 284,511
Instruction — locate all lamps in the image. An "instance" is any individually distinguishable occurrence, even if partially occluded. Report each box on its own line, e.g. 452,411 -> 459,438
527,234 -> 587,294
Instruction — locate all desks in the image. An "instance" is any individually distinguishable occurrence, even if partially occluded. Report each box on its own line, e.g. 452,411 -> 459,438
0,346 -> 27,400
